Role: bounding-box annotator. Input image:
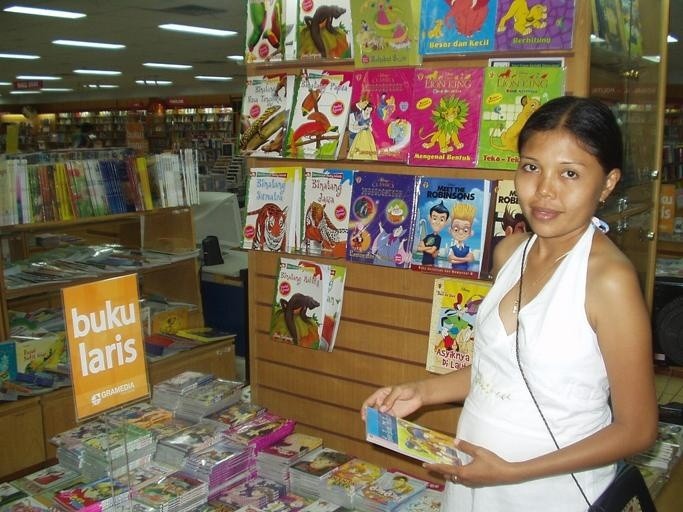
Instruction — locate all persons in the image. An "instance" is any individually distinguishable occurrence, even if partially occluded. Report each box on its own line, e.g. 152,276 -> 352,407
361,96 -> 657,512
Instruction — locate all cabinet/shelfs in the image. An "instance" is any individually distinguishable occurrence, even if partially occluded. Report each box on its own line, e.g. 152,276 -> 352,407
0,94 -> 243,480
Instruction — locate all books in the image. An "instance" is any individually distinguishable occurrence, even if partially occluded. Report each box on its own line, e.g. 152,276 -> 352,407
625,95 -> 683,497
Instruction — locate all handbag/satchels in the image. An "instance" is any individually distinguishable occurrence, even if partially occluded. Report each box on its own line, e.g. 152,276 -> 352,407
586,463 -> 658,512
